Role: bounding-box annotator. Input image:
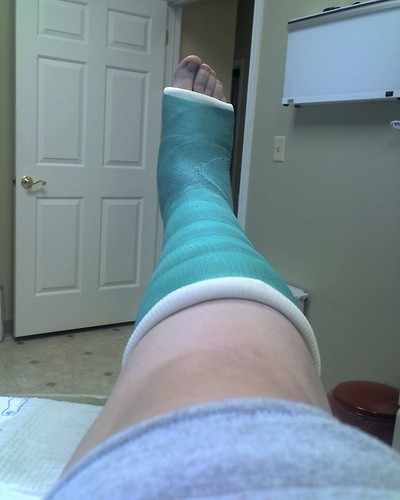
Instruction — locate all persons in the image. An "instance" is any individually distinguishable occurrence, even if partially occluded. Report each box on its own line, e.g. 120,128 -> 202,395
46,54 -> 400,500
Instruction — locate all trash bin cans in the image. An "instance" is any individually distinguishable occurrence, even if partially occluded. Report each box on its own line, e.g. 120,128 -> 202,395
329,381 -> 400,446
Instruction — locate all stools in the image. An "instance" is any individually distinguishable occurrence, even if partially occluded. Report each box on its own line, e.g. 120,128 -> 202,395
327,380 -> 400,446
